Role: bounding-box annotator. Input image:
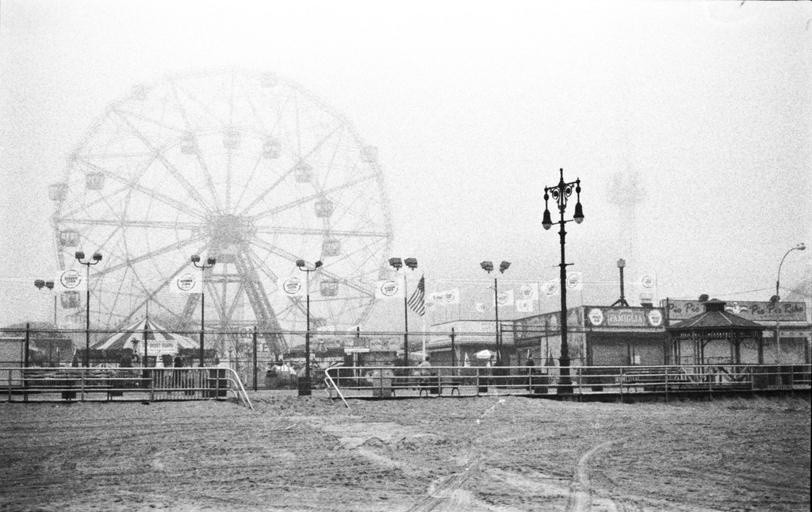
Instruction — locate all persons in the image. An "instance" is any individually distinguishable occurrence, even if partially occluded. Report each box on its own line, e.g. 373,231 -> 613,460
486,355 -> 497,390
266,360 -> 311,387
524,354 -> 537,391
166,349 -> 190,395
417,357 -> 432,396
413,361 -> 423,381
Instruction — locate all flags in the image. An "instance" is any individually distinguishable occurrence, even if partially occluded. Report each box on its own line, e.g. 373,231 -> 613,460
168,269 -> 203,295
426,270 -> 586,314
52,266 -> 88,294
407,277 -> 425,316
635,269 -> 658,293
374,274 -> 406,301
277,273 -> 308,298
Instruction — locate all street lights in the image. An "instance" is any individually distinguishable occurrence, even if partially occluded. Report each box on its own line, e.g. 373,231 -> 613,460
611,259 -> 629,307
190,255 -> 217,367
295,259 -> 323,379
479,259 -> 512,389
74,252 -> 102,394
542,168 -> 584,396
389,256 -> 418,389
775,242 -> 807,385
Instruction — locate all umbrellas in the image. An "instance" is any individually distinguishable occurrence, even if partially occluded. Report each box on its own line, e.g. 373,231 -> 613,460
472,350 -> 498,361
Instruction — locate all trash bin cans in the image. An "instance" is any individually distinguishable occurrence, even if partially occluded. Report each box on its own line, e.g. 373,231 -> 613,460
590,370 -> 603,391
479,378 -> 487,392
430,377 -> 442,394
753,363 -> 804,387
534,373 -> 548,393
298,377 -> 311,395
107,375 -> 123,396
209,369 -> 226,397
62,374 -> 76,399
